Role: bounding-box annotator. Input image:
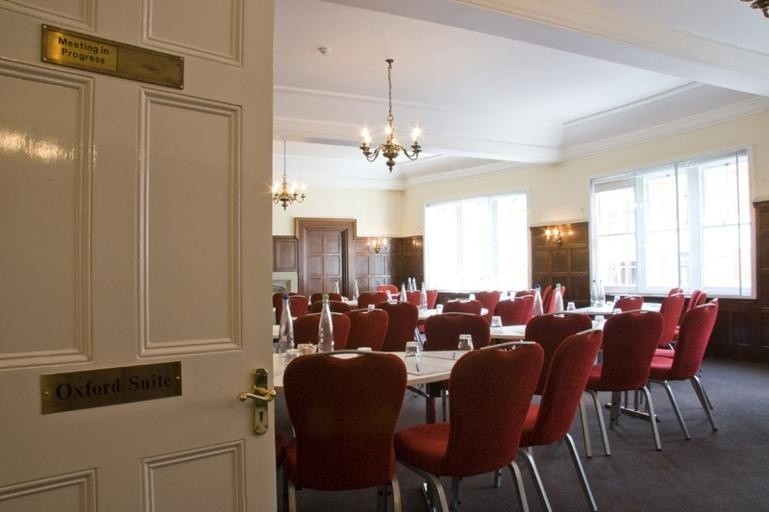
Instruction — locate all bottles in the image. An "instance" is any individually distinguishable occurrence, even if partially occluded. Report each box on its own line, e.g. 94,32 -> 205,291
411,278 -> 417,292
550,283 -> 564,317
333,278 -> 340,296
399,282 -> 407,303
352,279 -> 360,305
418,281 -> 428,316
278,289 -> 294,362
407,278 -> 413,291
532,284 -> 545,319
319,294 -> 334,355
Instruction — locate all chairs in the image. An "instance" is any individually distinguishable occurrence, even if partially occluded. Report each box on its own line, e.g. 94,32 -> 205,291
394,341 -> 545,512
584,309 -> 664,456
493,329 -> 603,512
276,350 -> 407,510
624,297 -> 718,440
525,313 -> 599,458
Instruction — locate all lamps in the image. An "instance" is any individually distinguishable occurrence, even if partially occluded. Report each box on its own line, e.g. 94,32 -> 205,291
358,59 -> 423,172
366,237 -> 388,255
271,140 -> 307,210
545,229 -> 562,244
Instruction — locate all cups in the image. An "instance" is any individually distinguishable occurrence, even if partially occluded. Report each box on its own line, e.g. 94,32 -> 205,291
404,342 -> 419,371
613,293 -> 620,305
567,301 -> 576,313
490,315 -> 504,333
367,304 -> 375,312
594,314 -> 605,331
385,289 -> 393,301
468,294 -> 476,301
458,334 -> 474,356
436,303 -> 444,316
